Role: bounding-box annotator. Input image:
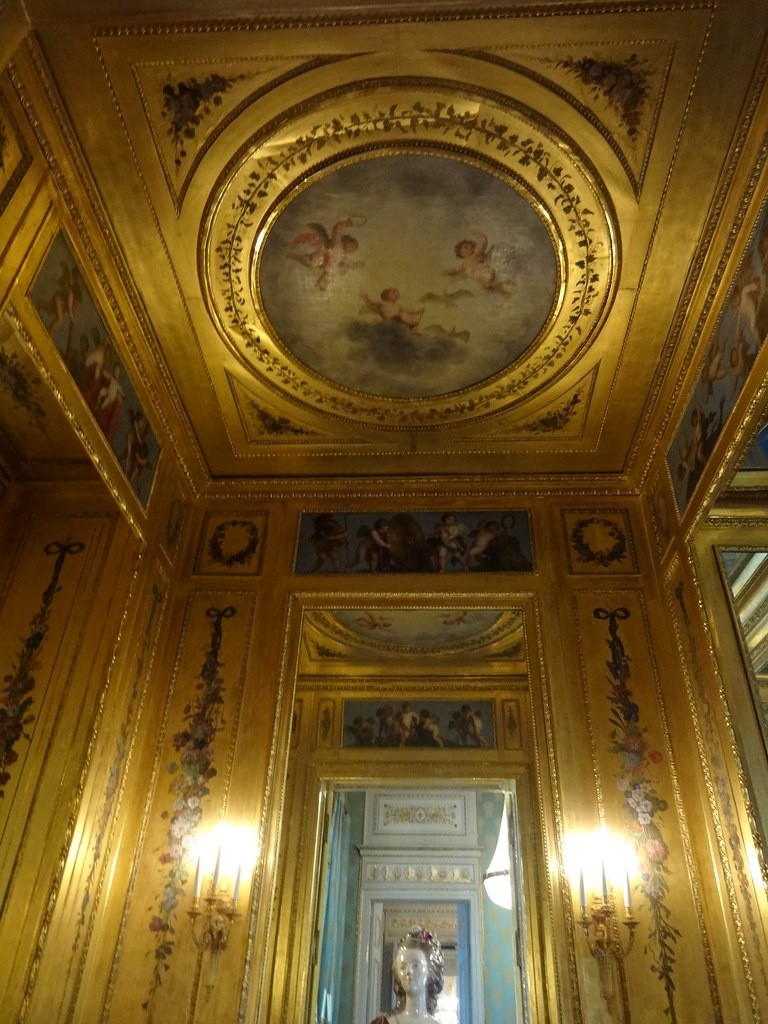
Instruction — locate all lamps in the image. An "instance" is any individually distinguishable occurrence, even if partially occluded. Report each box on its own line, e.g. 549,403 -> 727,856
563,824 -> 642,1015
185,818 -> 259,1003
